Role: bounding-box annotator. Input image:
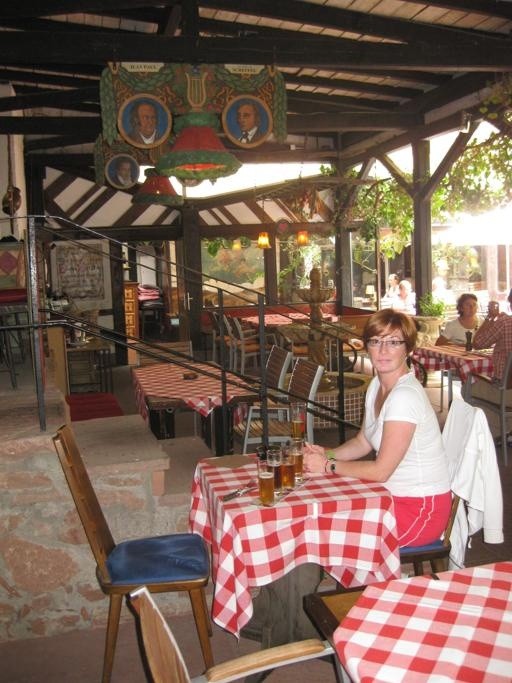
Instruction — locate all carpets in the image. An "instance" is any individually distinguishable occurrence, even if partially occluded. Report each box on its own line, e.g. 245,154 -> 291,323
66,393 -> 124,422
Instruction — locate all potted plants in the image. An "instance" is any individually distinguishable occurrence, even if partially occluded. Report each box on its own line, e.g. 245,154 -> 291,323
415,291 -> 447,334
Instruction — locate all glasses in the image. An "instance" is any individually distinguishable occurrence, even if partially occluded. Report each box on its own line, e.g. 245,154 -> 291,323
366,338 -> 407,348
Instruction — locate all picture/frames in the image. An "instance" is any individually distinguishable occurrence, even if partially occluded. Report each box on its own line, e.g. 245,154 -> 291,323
0,241 -> 26,289
104,152 -> 140,190
221,94 -> 274,149
117,94 -> 173,149
50,239 -> 112,310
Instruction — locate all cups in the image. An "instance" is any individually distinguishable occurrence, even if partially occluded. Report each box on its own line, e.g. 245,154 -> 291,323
254,438 -> 306,508
290,402 -> 307,441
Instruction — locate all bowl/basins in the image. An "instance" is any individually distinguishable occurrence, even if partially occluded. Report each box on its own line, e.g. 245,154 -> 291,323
362,301 -> 372,307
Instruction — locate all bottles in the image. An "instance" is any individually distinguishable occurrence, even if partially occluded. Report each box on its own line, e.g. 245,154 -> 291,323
465,329 -> 473,351
74,321 -> 87,344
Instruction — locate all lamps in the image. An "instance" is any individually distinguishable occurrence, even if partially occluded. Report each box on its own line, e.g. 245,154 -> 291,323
130,169 -> 184,207
157,110 -> 242,180
257,199 -> 271,249
296,190 -> 308,246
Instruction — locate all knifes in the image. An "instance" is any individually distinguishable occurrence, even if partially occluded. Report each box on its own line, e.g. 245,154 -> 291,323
221,487 -> 257,502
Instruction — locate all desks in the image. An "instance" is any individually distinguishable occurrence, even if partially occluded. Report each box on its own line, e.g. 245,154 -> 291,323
124,281 -> 140,339
65,332 -> 114,396
0,301 -> 28,388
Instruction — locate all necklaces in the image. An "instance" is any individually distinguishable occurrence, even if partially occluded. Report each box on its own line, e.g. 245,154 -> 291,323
461,317 -> 475,323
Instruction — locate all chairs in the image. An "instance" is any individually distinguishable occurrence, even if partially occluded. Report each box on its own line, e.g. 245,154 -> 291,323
131,585 -> 352,683
439,320 -> 460,412
51,424 -> 215,683
336,396 -> 505,590
134,309 -> 373,455
461,351 -> 512,468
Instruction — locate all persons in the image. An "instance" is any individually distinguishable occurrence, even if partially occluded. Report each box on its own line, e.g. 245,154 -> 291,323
302,309 -> 452,549
461,288 -> 512,447
235,104 -> 263,144
114,160 -> 135,186
391,279 -> 416,312
435,293 -> 486,347
127,102 -> 167,143
385,274 -> 401,297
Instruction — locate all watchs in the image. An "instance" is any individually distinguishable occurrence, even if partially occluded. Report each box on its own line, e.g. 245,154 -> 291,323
331,459 -> 337,475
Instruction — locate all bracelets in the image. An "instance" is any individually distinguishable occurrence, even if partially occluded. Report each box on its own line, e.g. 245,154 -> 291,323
485,315 -> 494,322
325,457 -> 334,473
326,448 -> 336,459
